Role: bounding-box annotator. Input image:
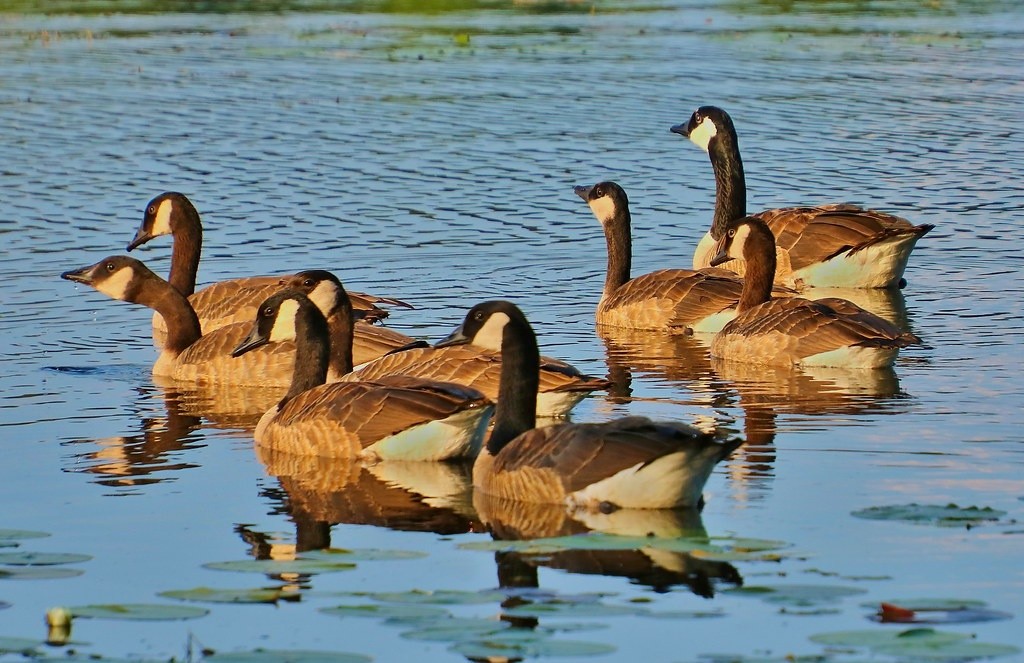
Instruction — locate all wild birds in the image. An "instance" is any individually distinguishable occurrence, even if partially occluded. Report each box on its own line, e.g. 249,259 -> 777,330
229,290 -> 496,462
53,377 -> 201,498
570,181 -> 798,333
594,323 -> 934,498
434,299 -> 747,509
283,270 -> 615,417
228,442 -> 498,605
668,106 -> 935,285
125,190 -> 416,335
57,256 -> 436,387
461,488 -> 744,663
707,216 -> 937,372
